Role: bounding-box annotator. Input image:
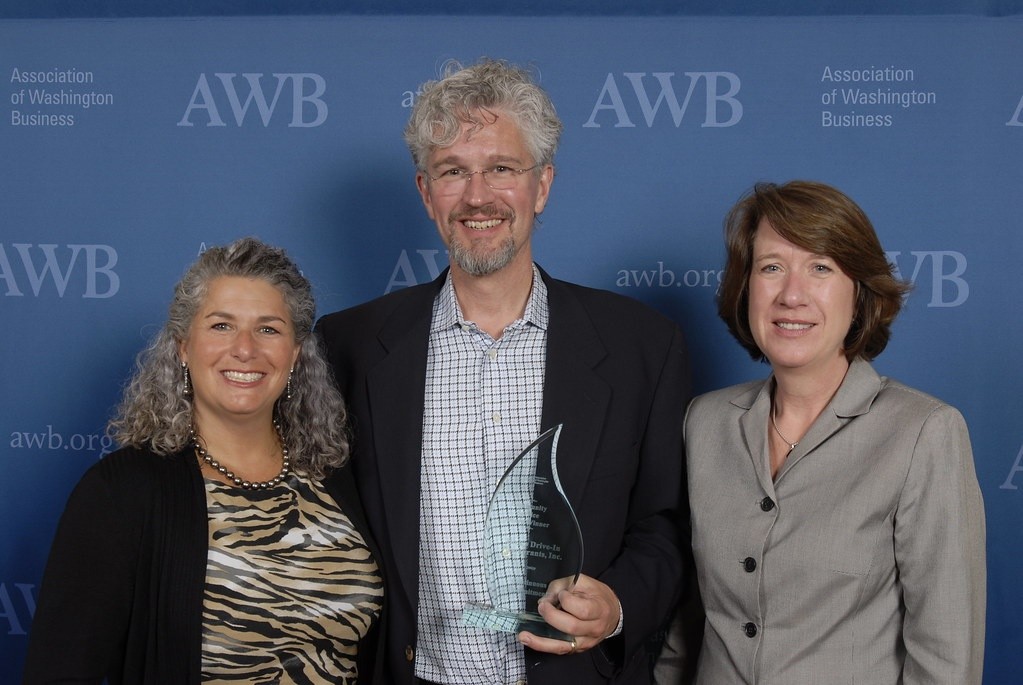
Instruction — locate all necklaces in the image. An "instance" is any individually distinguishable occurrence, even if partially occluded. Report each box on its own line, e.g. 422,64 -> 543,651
189,417 -> 290,488
772,387 -> 799,459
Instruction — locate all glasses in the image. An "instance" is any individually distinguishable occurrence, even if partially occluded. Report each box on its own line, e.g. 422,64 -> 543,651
420,157 -> 545,191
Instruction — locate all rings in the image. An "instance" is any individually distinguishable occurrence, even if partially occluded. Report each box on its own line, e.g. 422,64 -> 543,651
569,639 -> 577,654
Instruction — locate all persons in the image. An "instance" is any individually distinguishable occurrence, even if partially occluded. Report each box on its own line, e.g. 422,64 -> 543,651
646,180 -> 986,685
311,62 -> 696,685
20,238 -> 386,685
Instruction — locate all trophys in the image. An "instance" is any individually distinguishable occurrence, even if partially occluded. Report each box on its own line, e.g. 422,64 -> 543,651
461,423 -> 584,644
